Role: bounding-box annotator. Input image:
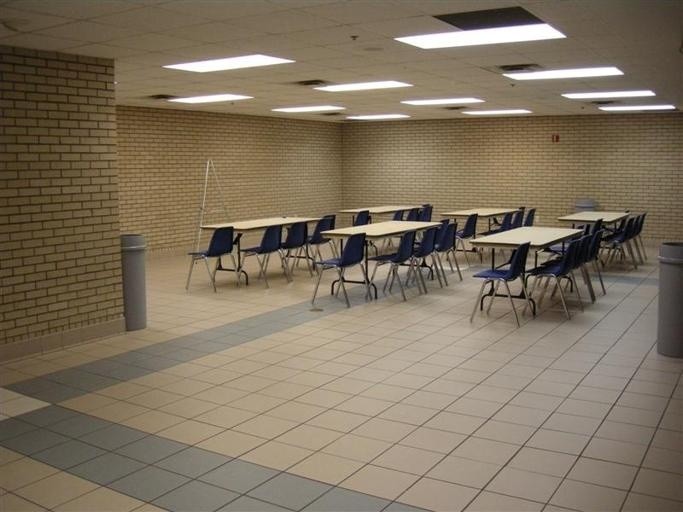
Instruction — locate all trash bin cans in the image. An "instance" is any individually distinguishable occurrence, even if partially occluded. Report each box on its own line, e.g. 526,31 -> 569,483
658,242 -> 683,357
120,234 -> 147,331
575,198 -> 595,213
